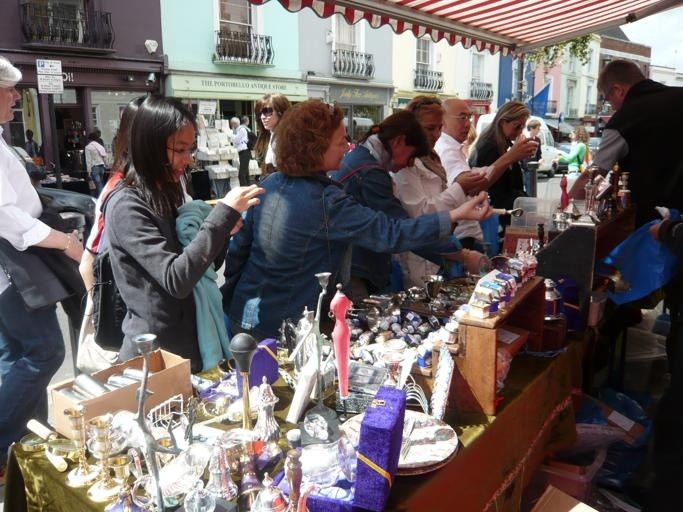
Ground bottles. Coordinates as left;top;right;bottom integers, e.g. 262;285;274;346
585;168;598;213
545;276;562;322
56;316;337;511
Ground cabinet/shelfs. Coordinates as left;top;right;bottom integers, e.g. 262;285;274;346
12;196;636;512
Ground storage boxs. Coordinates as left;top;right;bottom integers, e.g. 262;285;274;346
621;323;669;395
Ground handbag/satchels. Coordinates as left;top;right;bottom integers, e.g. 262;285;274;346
90;182;134;352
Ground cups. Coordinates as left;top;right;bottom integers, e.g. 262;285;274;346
618;189;630;209
526;141;540;159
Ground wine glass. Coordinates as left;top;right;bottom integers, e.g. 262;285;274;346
420;274;446;313
377;336;408;386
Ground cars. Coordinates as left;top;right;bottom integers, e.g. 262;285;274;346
34;185;98;242
475;114;608;177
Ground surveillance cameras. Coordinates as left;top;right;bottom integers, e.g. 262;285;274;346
147;73;156;84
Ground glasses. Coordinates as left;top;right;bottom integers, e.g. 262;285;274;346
510;120;526;131
166;146;198;161
259;106;281;117
